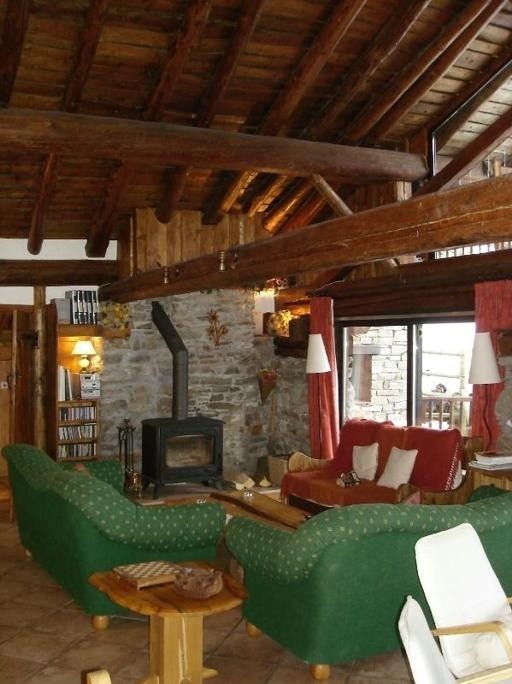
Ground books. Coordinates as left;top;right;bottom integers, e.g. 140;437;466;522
336;471;361;488
59;406;97;458
112;560;183;591
468;451;512;471
58;365;73;401
65;290;96;324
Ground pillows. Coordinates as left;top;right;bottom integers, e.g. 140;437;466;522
352;442;380;481
375;446;419;490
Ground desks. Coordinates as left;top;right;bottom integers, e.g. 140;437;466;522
86;560;246;684
165;488;315;533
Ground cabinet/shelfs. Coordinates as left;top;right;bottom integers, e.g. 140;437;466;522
45;303;104;464
466;463;512;491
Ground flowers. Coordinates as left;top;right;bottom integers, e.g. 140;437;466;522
256;368;281;402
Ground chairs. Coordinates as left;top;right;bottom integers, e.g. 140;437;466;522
398;593;512;684
415;523;512;679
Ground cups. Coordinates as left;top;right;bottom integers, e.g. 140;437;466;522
242;487;255;505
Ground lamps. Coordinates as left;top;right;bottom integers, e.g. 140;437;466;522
71;340;96;374
468;331;512;464
161;265;181;284
306;333;332;460
217;249;238;273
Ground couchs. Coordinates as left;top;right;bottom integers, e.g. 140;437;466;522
1;442;225;628
281;418;483;508
225;485;511;681
